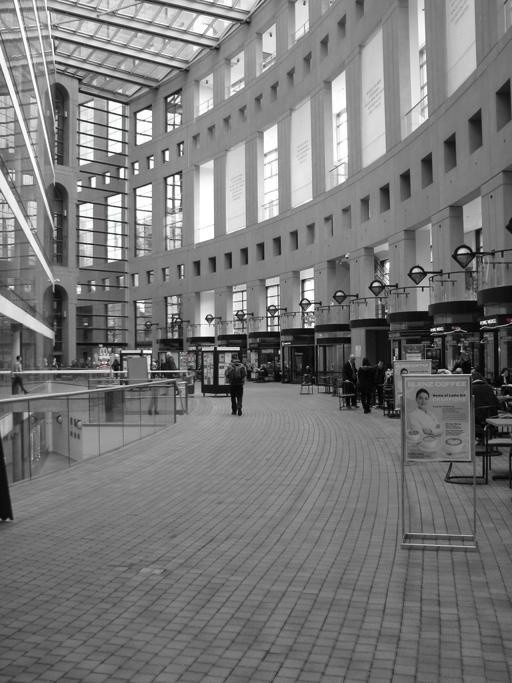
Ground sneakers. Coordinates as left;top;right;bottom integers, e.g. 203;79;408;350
345;404;360;411
231;407;243;416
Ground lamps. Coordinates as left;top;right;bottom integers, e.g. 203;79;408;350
144;321;159;329
266;290;370;318
174;310;264;327
367;244;512;298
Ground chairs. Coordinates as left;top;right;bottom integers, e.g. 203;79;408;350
295;359;511;487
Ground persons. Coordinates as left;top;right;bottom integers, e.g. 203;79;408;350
224;355;247;415
163;350;180;395
410;387;438;432
357;356;376;414
147;358;161;415
260;365;268;376
112;358;121;379
371;359;385;407
342;354;359;408
454;365;511;446
11;354;30;394
395;367;409;394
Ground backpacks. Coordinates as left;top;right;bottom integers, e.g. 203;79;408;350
228;362;242;380
